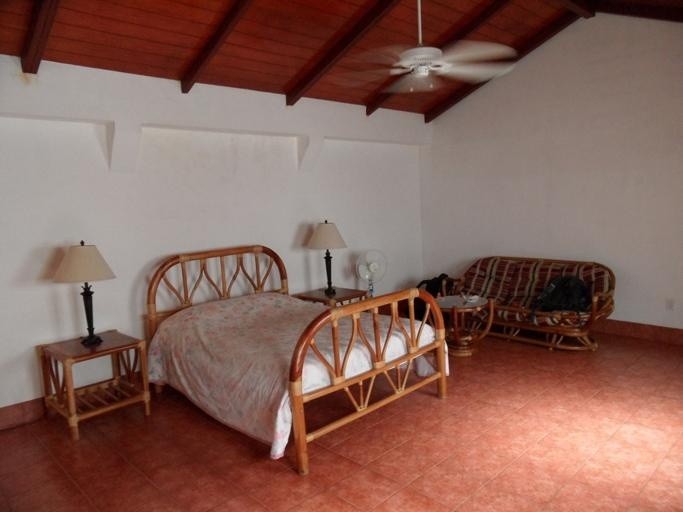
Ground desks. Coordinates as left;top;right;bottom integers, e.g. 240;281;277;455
34;327;153;441
289;286;369;308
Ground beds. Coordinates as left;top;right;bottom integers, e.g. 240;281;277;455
142;243;453;478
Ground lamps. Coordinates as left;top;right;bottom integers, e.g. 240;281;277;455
51;239;118;346
305;219;350;294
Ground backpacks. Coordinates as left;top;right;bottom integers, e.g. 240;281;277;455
530;275;589;326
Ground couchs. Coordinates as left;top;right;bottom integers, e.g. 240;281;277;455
439;254;618;353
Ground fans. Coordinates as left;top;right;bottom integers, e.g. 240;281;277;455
322;3;518;95
354;246;388;300
